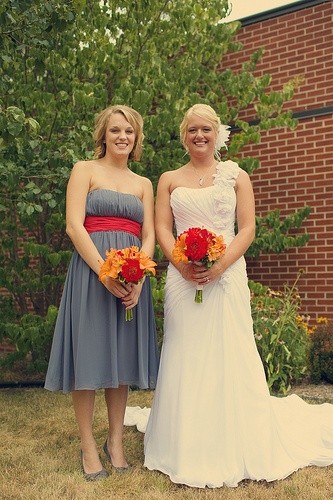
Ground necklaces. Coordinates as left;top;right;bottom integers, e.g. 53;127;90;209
187;161;218;186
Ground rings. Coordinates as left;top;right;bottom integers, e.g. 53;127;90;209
205;277;208;281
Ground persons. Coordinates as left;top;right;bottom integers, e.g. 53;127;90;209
44;103;158;480
144;103;271;490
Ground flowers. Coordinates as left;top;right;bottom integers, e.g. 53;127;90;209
172;228;227;304
97;246;157;322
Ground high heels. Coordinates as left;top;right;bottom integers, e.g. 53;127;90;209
104;442;129;472
80;446;108;482
